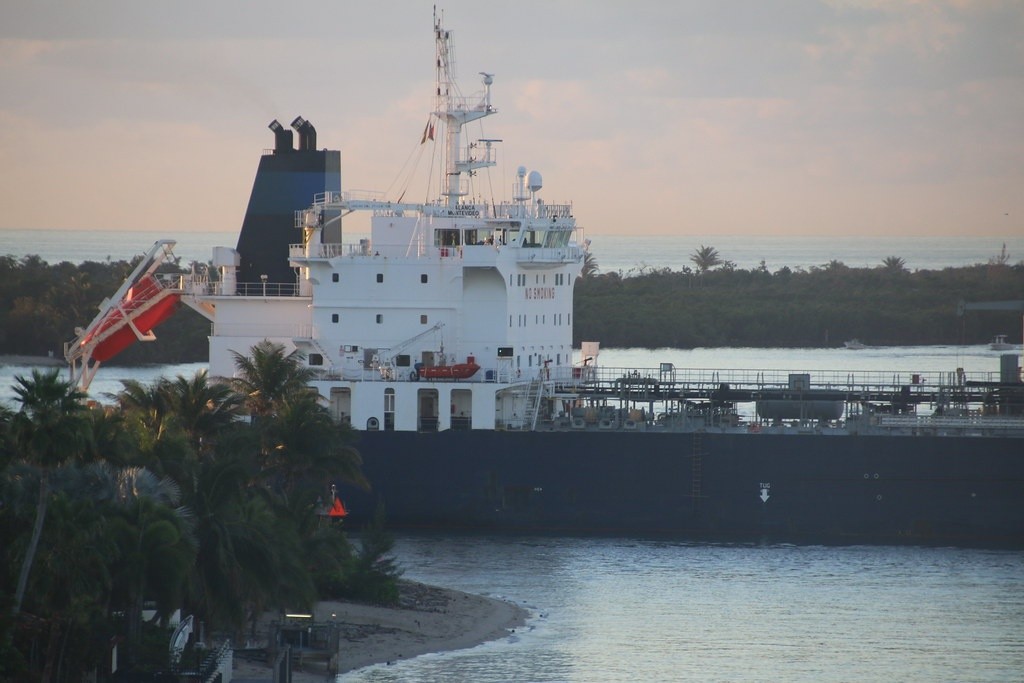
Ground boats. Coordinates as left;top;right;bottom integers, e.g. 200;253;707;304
844;339;864;349
62;3;1023;546
990;336;1016;351
